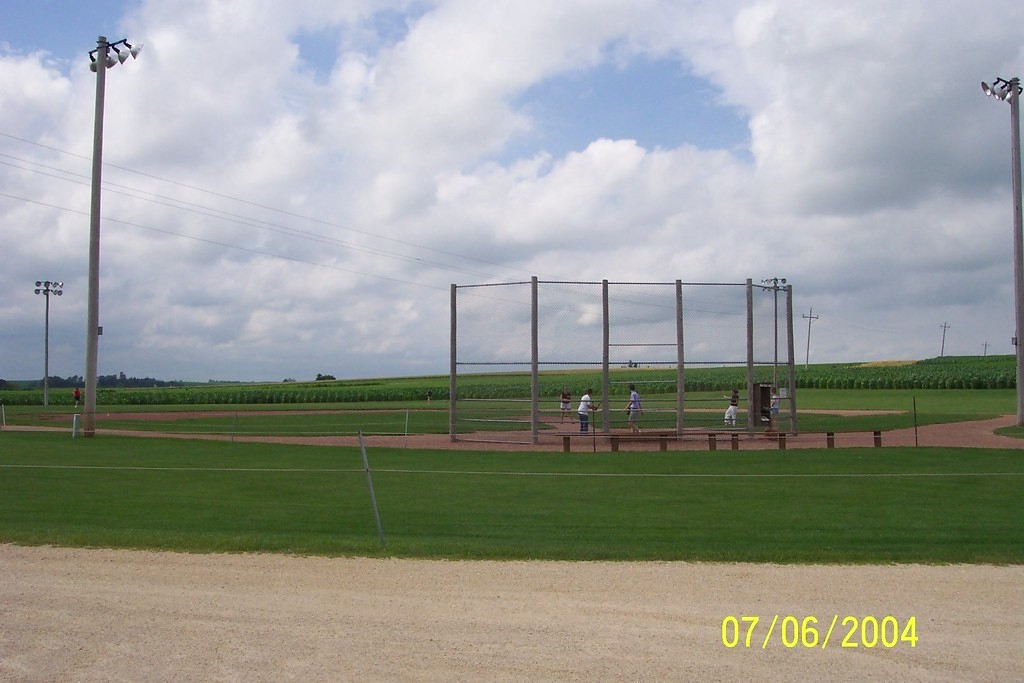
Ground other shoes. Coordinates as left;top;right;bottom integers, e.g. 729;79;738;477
764;428;772;432
770;428;779;432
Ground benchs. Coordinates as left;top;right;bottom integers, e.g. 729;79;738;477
554;429;888;452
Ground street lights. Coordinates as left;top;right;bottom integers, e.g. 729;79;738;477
83;34;145;439
34;280;64;406
982;76;1024;428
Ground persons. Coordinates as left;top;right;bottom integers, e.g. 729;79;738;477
560;386;575;424
578;389;597;432
72;387;81;408
770;386;779;431
724;388;739;426
624;383;643;432
